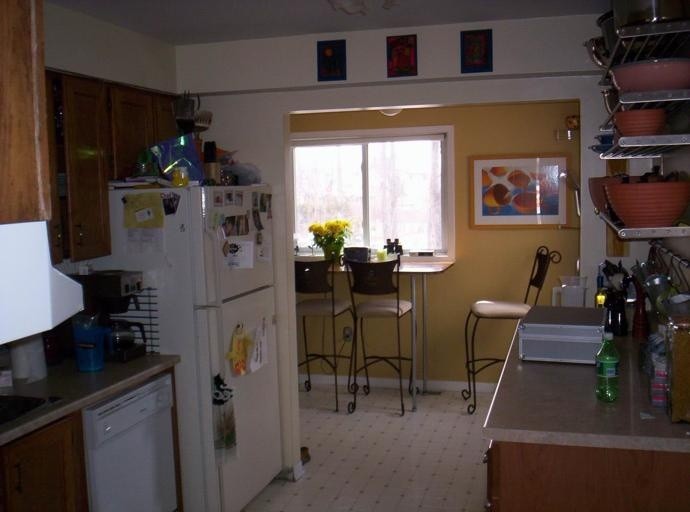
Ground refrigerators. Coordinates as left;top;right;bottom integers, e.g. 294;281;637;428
109;178;284;511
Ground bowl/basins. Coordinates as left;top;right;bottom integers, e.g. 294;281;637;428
612;111;668;136
587;174;689;227
588;134;613;152
583;0;689;115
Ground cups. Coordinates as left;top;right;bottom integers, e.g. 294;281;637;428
376;250;387;261
641;270;690;314
204;162;221;184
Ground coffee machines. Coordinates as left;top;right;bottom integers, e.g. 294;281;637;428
92;270;146;362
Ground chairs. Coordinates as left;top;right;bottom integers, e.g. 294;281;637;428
459;245;563;415
295;253;416;416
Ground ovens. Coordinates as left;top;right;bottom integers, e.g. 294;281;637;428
79;374;179;512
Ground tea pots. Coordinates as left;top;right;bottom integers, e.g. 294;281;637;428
552;275;588;308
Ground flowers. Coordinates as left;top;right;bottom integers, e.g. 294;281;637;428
308;218;351;247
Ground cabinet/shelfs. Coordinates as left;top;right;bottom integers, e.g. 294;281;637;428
109;82;175;185
482;439;688;512
42;71;111;266
1;410;91;512
598;20;689;242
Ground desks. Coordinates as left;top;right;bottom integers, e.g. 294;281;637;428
296;258;455;394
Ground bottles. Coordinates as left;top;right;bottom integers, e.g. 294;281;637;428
594;333;620;403
595;277;628;334
172;166;188;187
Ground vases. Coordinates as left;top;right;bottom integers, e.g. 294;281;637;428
323;241;343;265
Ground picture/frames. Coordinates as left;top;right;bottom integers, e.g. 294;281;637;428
468;151;571;229
